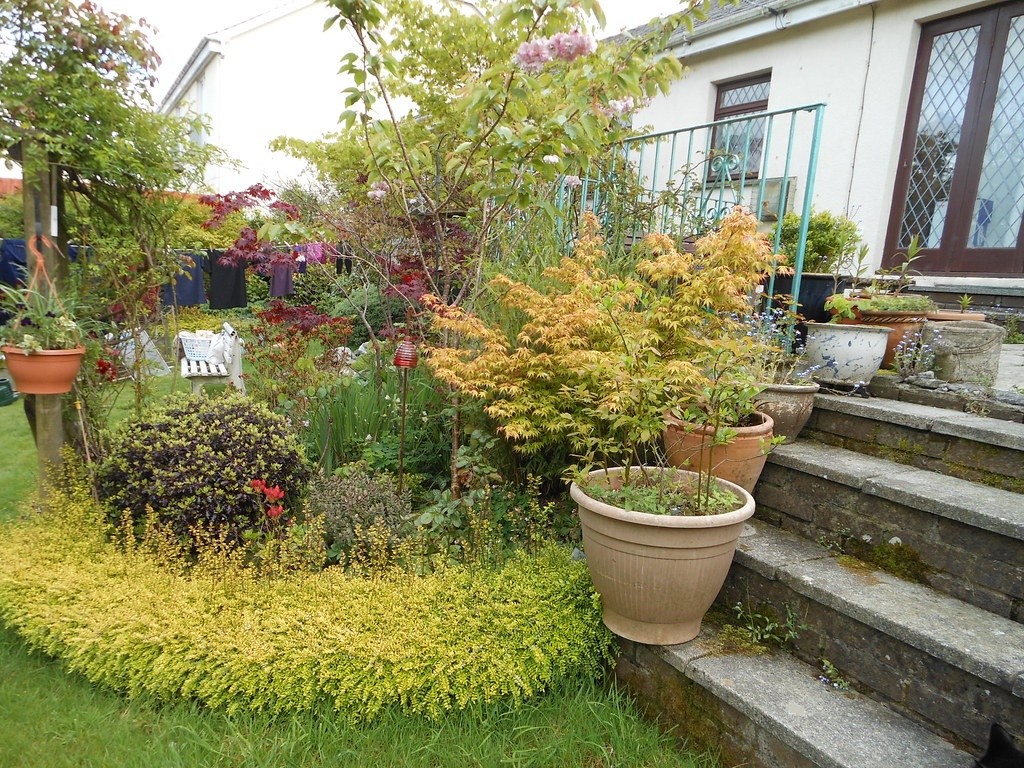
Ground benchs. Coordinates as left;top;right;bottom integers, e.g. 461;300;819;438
179;322;246;396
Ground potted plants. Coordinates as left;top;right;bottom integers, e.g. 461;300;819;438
422;207;985;646
0;314;85;395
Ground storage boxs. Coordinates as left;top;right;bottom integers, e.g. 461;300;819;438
182;336;210;362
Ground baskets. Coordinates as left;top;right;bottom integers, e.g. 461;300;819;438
179;331;212;360
